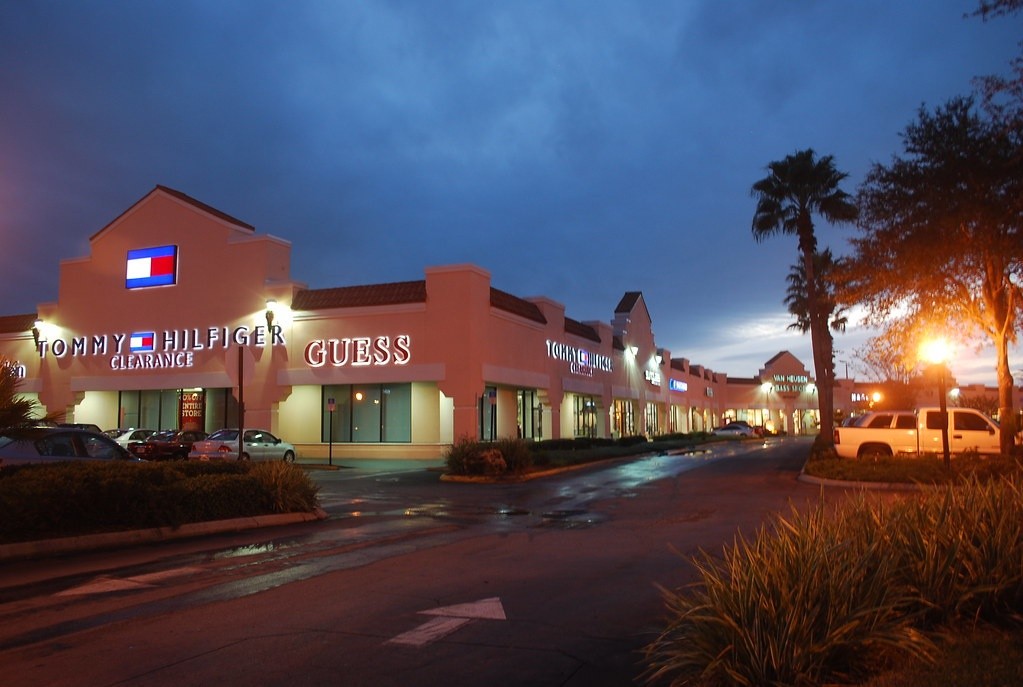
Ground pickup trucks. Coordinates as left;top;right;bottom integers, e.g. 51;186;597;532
833;405;1023;465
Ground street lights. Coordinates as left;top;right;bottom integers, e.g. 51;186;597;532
919;335;954;468
838;359;849;380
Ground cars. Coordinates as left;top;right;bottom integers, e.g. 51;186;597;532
841;408;918;428
709;423;755;439
84;426;157;458
0;425;149;466
186;427;297;465
127;427;211;462
55;421;102;434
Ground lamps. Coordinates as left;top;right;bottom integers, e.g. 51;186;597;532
266;298;277;333
31;319;47;347
